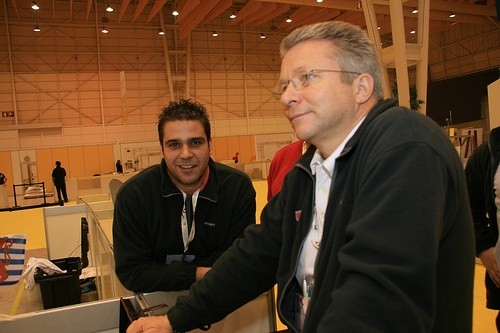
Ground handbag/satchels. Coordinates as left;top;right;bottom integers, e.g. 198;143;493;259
0;234;26;286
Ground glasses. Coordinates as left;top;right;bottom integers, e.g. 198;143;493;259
272;68;362;100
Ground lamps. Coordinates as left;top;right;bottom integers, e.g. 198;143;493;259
212;31;219;37
158;0;180;35
102;1;114;33
31;0;42;32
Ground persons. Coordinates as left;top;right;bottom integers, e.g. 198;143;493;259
0;173;9;209
267;139;310;202
112;98;257;333
126;20;474;333
453;130;477;158
116;160;123;174
465;126;500;333
52;161;69;206
235;151;239;163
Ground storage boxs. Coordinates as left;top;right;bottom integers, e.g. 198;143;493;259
36;216;89;310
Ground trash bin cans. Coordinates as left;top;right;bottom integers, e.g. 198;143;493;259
34;257;82;309
